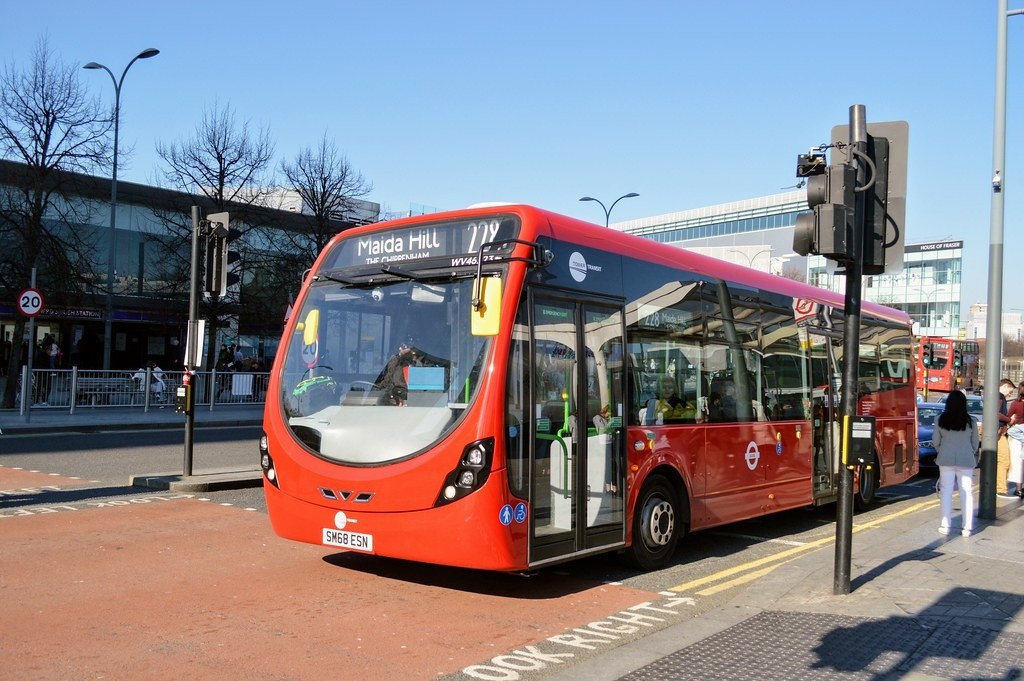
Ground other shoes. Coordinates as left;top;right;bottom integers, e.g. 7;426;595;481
962;529;971;538
155;394;160;400
159;405;165;408
43;401;50;407
938;527;951;535
1014;488;1024;500
997;492;1019;499
50;373;57;376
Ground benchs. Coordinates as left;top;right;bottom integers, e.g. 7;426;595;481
76;378;180;410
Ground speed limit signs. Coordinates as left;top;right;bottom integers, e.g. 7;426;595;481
17;288;44;316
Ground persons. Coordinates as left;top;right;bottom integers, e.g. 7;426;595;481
668;361;676;375
933;390;979;537
639;399;663;425
813;403;826;472
382;333;424;399
765;396;792;419
593;404;611;434
568;411;577;454
650;359;655;370
708;391;722;418
216;343;261;402
998;379;1024;499
656;376;686;409
33;333;58;406
136;361;168;409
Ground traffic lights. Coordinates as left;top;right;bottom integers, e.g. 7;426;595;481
207;212;242;298
954;349;961;366
922;344;931;365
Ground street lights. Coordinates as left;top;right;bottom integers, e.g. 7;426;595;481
82;47;159;376
579;193;640;228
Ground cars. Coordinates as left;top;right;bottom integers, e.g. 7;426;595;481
917;402;946;469
936;394;983;441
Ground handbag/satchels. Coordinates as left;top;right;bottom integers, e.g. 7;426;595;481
978;425;983;441
45;346;51;356
227;362;234;367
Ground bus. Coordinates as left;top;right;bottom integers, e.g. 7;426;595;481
260;205;919;577
916;337;979;391
